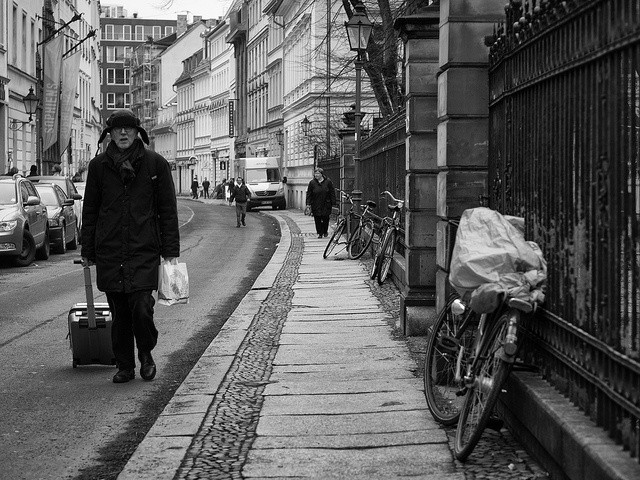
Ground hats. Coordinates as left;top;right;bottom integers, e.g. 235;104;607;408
98;108;150;146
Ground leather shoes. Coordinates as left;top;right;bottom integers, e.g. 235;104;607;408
140;353;156;380
113;369;134;383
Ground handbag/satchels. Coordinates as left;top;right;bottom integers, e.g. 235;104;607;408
158;258;190;307
304;205;312;216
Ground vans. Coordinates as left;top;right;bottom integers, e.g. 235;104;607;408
231;157;288;212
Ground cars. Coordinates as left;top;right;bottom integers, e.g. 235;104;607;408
0;173;50;267
73;180;87;204
27;172;86;253
30;182;79;255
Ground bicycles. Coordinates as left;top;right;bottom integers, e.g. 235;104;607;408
368;190;405;286
420;203;550;461
322;187;377;262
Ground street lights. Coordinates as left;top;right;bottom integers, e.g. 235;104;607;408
59;27;100;165
342;2;376;265
33;9;84;171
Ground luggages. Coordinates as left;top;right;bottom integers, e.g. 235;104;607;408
68;260;117;368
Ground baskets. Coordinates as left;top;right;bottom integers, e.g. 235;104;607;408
448;219;461;274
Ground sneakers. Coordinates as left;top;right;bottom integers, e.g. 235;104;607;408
241;220;245;226
318;235;322;238
237;224;240;227
324;233;328;237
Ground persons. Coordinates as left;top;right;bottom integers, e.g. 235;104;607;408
305;167;336;239
72;171;83;182
202;177;210;199
6;167;19;176
26;164;38;177
230;177;251;228
51;162;63;177
190;178;199;199
80;109;181;383
